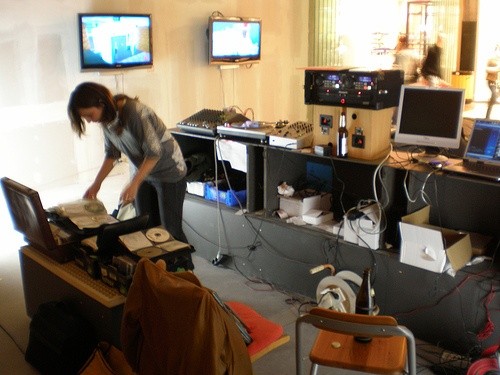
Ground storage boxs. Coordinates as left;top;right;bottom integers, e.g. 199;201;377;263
302;208;334;225
186;170;230;197
277;187;331;219
343;202;383;251
204;182;247;208
398;204;472;274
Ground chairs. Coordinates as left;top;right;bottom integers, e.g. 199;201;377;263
295;307;416;375
119;256;291;375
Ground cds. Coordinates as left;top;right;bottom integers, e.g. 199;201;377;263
147;228;169;242
138;247;161;259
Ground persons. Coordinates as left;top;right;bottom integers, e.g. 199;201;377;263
68;81;195;274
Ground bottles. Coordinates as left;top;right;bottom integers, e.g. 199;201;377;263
336;112;349;158
354;270;373;344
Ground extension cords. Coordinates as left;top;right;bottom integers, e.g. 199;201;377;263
440;352;473;369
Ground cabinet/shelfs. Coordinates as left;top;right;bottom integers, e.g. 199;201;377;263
169;123;500;358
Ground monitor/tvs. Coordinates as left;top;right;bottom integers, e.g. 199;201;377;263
209;17;263;64
463;120;500;170
394;86;465;162
77;13;154;72
